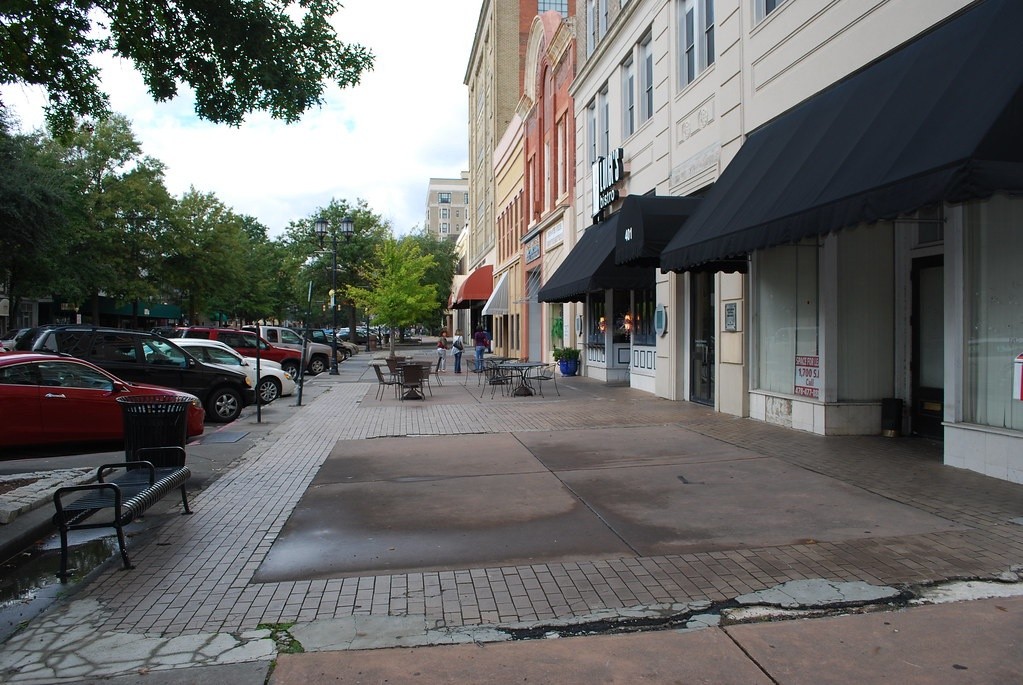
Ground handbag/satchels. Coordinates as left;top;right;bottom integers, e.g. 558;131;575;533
437;337;444;349
484;339;487;347
453;336;463;350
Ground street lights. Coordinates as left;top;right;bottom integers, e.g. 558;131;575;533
314;217;355;375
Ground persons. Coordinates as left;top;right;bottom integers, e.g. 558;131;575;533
436;330;449;373
474;326;491;373
451;329;465;374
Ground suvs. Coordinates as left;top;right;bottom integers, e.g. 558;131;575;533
11;323;256;424
166;325;302;382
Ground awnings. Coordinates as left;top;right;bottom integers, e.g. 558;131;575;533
538;211;620;303
448;265;494;309
615;194;703;263
481;272;507;316
75;295;184;321
660;0;1023;276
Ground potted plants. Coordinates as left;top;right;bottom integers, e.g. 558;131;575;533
553;347;581;378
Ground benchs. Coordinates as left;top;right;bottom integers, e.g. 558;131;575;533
55;446;194;585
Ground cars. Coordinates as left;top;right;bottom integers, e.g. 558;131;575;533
284;326;359;360
0;350;206;447
336;326;383;345
125;337;295;405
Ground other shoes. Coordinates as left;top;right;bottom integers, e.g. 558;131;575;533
438;369;445;372
475;369;483;373
455;370;461;373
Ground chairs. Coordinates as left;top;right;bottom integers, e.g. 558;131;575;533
464;356;560;402
372;357;442;403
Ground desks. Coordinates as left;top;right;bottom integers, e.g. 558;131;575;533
476;357;520;376
498;361;549;397
395;367;429;400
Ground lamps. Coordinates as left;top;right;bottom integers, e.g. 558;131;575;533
623;312;632;336
598;316;607;333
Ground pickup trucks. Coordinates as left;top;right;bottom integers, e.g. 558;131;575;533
241;324;342;376
